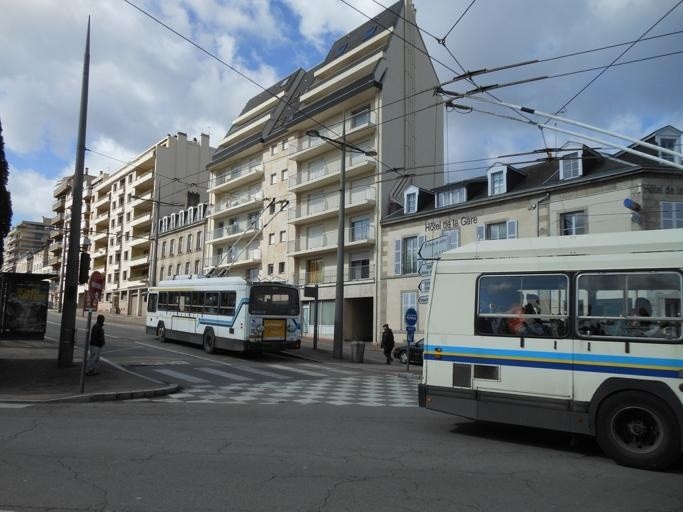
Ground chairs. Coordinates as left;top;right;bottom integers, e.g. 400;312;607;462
549;313;564;338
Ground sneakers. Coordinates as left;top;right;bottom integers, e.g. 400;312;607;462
85;371;99;377
384;358;394;365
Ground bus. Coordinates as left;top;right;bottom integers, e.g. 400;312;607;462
414;223;682;476
141;274;303;358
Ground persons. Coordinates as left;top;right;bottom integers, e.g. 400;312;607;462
488;289;670;338
378;321;398;365
85;313;106;378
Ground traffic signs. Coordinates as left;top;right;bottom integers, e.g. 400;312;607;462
416;233;450;306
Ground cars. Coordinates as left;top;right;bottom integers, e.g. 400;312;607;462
393;336;423;365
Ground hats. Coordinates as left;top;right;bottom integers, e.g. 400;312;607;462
382;324;388;327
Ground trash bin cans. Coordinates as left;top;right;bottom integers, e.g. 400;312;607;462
351;342;365;363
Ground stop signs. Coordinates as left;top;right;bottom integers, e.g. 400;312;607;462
87;270;103;301
